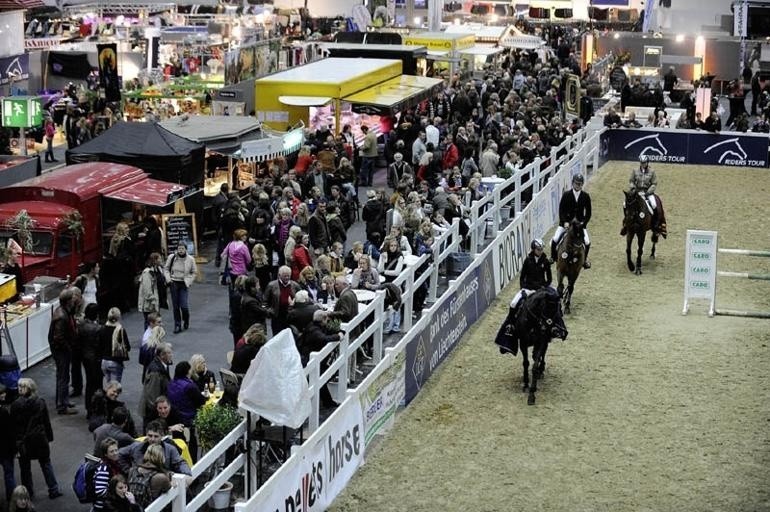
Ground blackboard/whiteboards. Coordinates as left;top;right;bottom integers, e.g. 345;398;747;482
161;213;199;258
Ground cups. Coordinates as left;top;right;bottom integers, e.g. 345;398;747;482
205;171;228;193
317;293;337;312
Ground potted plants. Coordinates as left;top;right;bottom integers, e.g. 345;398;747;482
493;165;515;220
193;402;244;510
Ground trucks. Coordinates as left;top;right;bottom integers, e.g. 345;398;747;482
1;160;205;299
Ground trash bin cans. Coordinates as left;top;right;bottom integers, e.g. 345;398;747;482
478;176;505;193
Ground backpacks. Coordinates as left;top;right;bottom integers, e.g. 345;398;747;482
128;467;157;507
72;457;98;504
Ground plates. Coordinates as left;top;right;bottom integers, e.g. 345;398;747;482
353;290;365;296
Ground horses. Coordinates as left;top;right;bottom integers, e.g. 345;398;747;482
555;220;586;315
517;287;569;405
622;189;665;276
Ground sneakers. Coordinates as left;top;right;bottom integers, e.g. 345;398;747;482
49;491;63;499
57;391;83;414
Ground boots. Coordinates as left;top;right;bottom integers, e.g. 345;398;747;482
548;240;556;264
583;243;591;268
182;308;190;329
173;309;181;333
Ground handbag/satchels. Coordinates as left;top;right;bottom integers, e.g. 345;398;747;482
115;329;130;362
220;266;232;286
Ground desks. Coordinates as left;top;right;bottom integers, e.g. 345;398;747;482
478;176;506;226
314;241;427;384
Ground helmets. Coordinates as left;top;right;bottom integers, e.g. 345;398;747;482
573;173;584;185
532;238;545;250
640;155;649;163
